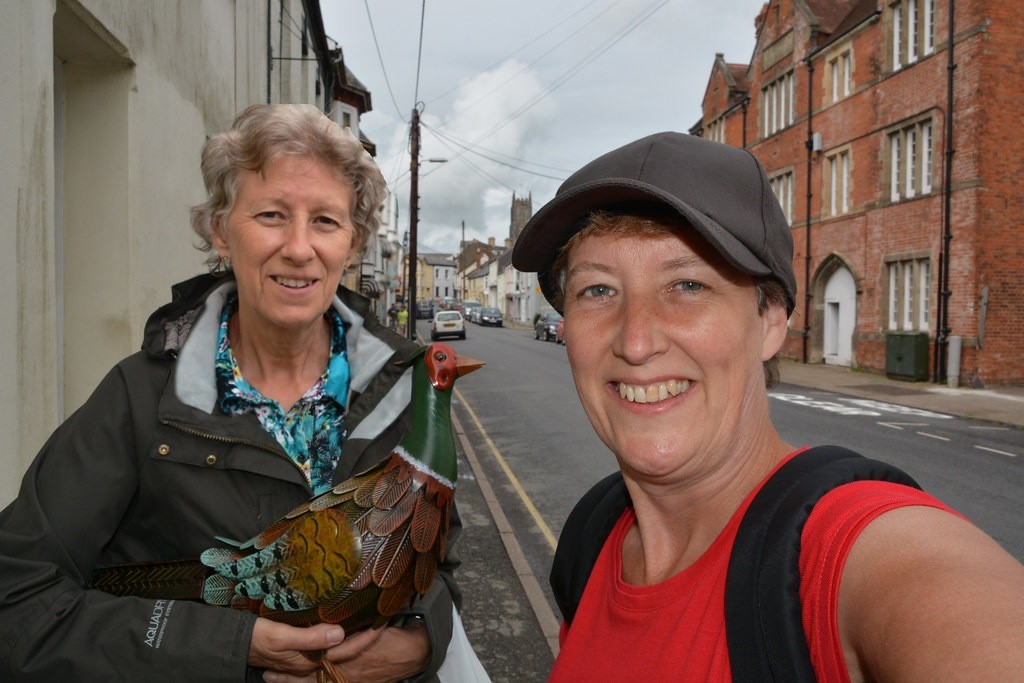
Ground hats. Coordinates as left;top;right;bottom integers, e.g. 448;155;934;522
510;129;797;319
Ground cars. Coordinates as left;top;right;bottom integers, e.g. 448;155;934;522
534;311;563;342
428;310;467;342
555;317;566;346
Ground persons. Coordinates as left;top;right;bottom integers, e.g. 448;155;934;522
388;304;399;332
0;103;465;683
398;305;408;337
511;132;1023;683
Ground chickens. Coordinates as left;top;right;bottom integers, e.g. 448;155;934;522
85;343;486;683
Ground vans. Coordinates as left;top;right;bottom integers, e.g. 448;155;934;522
461;299;481;320
480;307;504;328
470;307;481;324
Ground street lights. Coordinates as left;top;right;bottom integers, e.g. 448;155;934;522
408;158;450;341
